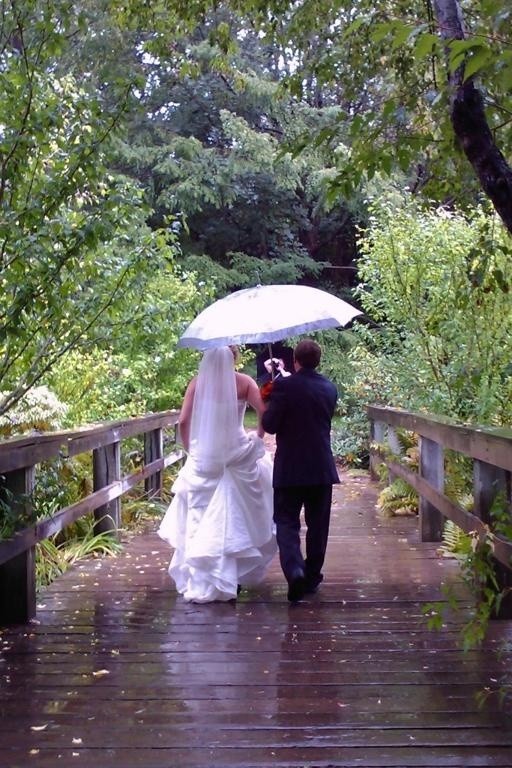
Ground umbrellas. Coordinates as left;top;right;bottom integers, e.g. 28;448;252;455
177;271;365;348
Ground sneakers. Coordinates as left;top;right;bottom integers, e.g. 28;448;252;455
288;571;323;604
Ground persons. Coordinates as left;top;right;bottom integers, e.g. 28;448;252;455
257;340;342;602
156;346;278;605
264;357;293;382
